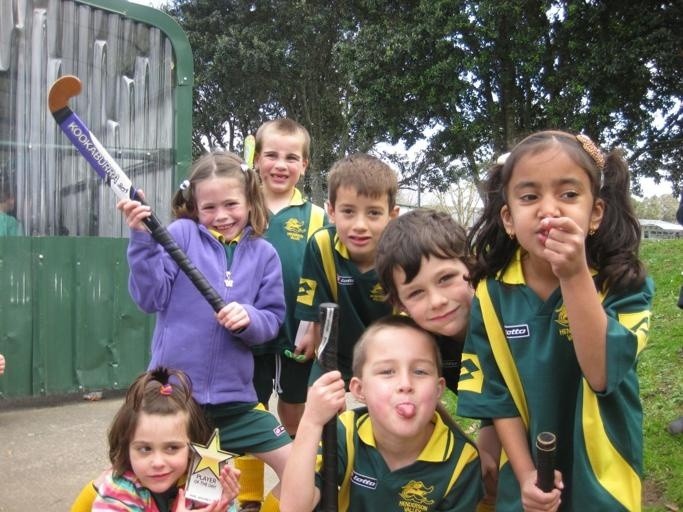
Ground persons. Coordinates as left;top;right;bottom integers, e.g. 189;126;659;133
666;193;683;435
278;313;489;512
373;208;502;512
454;129;656;512
233;115;332;512
90;363;242;512
292;154;401;396
0;354;6;375
71;150;293;512
0;188;24;237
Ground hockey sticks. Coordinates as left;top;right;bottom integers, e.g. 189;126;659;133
48;75;224;310
